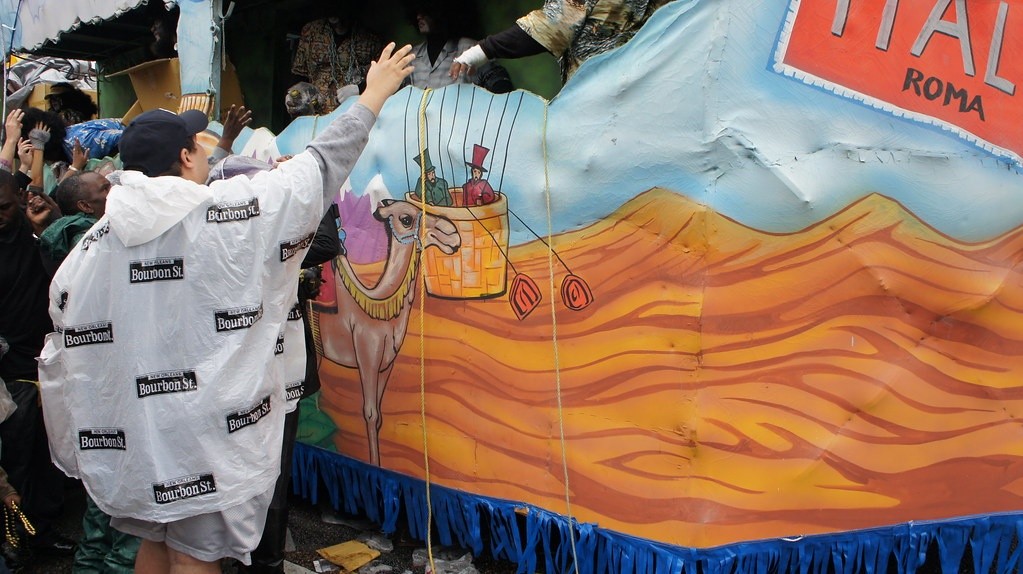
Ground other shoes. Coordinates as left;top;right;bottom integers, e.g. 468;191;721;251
49;536;79;557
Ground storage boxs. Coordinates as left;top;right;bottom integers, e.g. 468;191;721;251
29;84;98;119
105;57;245;127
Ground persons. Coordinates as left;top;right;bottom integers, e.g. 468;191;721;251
448;0;676;88
37;40;417;574
0;83;343;574
285;1;389;119
401;0;515;93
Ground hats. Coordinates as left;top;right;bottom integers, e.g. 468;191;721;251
117;109;209;178
44;81;71;100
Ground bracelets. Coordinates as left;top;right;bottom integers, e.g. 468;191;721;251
67;165;78;171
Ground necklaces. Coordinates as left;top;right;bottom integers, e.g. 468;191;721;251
326;21;360;88
3;499;36;548
